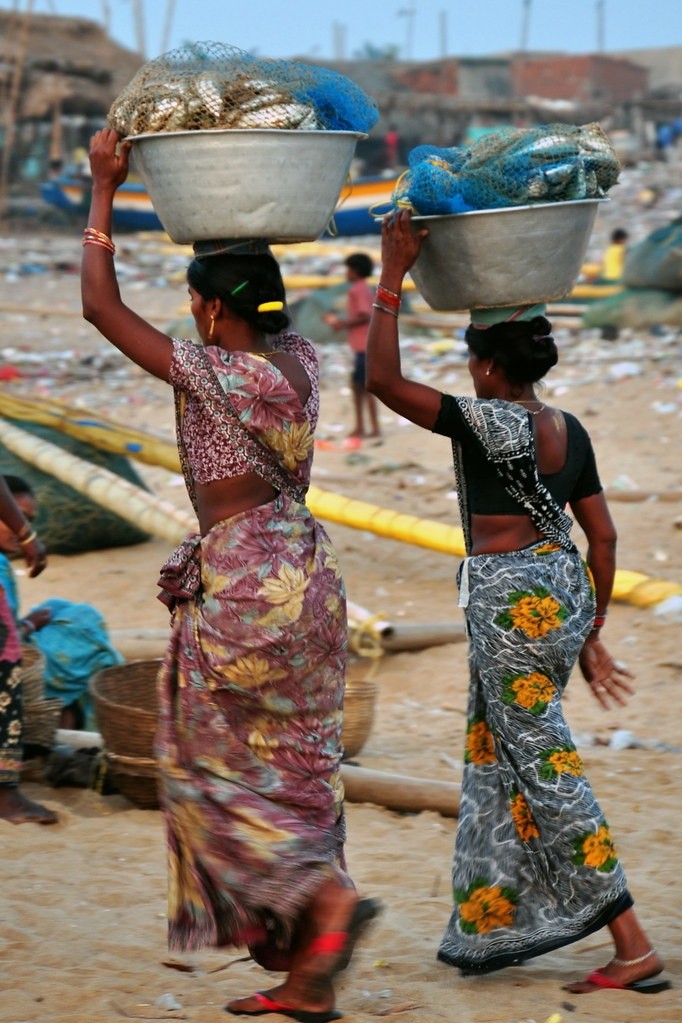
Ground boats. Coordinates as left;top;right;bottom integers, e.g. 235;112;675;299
38;159;414;240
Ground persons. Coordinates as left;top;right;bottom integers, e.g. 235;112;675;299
323;253;383;438
76;125;388;1023
599;228;628;281
365;205;671;998
0;474;83;783
0;458;60;826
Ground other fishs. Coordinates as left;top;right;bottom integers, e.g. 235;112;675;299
114;68;327;137
452;117;622;199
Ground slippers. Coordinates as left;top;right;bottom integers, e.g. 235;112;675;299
222;992;342;1023
308;896;378;974
563;967;671;995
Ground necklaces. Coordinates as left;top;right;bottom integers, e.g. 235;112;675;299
513;398;547;416
252;351;283;359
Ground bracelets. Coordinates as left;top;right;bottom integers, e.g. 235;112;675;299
81;227;116;256
592;609;607;630
372;284;401;318
16;519;40;548
22;619;37;631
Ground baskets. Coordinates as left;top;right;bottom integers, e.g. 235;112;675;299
87;660;169;809
15;697;62;778
339;678;376;760
17;643;46;703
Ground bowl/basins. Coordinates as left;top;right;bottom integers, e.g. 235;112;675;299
374;197;611;312
121;129;369;245
